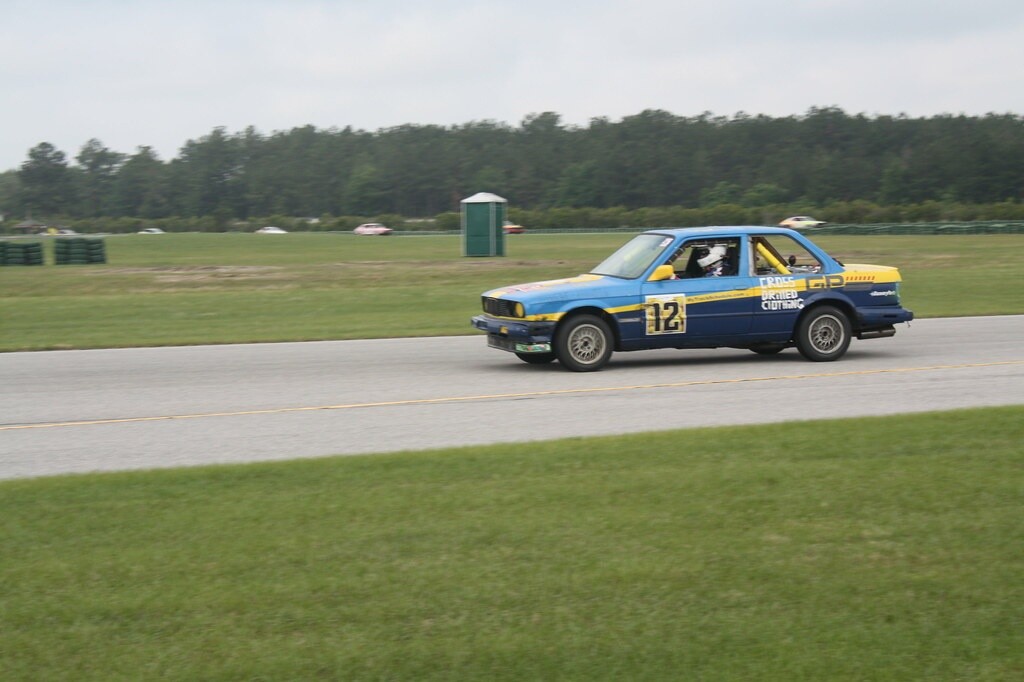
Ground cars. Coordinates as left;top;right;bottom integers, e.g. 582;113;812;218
354;222;393;235
470;225;916;374
254;227;288;234
503;221;524;235
137;227;165;235
778;216;828;229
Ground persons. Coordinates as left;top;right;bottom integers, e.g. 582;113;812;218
670;243;728;279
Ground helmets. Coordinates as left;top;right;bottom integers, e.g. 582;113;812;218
691;238;727;268
